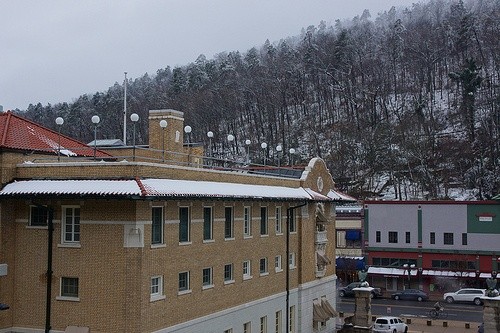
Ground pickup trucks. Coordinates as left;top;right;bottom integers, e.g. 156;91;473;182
337;281;384;299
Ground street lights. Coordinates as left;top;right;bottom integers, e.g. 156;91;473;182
207;131;214;166
55;116;64;162
130;113;139;161
91;115;100;161
276;145;283;174
245;140;251;165
159;120;167;163
289;148;295;169
184;125;192;166
227;135;234;172
261;142;268;175
403;263;415;289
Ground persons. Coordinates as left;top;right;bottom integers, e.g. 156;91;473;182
434;301;442;317
478;323;484;333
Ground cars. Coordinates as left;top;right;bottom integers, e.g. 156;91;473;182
391;289;429;302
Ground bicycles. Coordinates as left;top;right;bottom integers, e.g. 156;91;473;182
430;308;448;317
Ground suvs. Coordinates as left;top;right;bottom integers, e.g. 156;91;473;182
372;317;408;333
443;288;488;306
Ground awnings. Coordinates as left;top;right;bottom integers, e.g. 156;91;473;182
336;255;365;271
